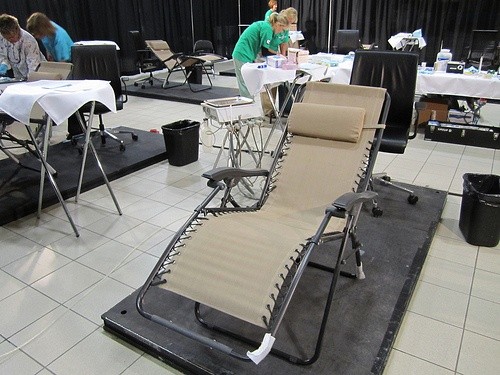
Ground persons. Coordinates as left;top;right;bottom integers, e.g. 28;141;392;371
264;0;279;21
232;7;298;118
26;13;88;141
0;13;47;81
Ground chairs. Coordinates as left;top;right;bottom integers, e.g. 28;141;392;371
333;29;361;53
27;60;73;82
146;40;229;94
134;78;396;366
68;43;138;152
195;40;219;79
134;49;169;89
350;49;422;215
464;29;500;72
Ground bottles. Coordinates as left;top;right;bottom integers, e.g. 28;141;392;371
437;49;453;62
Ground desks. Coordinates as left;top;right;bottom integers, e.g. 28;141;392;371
388;32;428;53
0;78;123;237
412;67;500;125
240;60;334;155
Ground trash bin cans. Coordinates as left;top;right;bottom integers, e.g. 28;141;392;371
161;120;200;167
185;66;202;84
459;173;500;247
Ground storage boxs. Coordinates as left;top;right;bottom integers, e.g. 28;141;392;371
286;47;309;65
446;61;466;74
415;101;449;126
267;55;285;69
